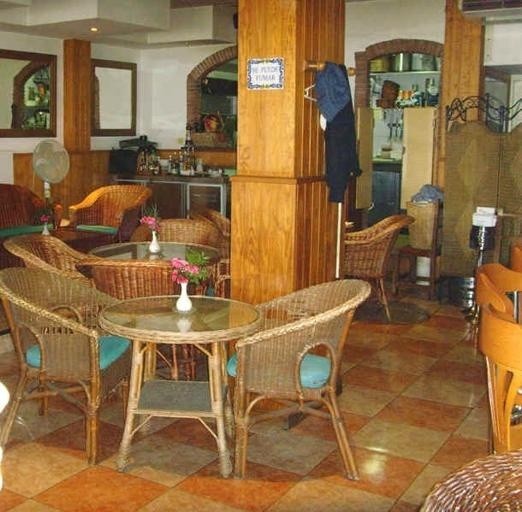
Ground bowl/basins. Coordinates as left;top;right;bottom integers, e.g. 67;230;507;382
375;79;400;108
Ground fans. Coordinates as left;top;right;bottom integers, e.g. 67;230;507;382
32;139;70;199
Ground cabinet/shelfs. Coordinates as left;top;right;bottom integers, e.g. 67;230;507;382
350;38;444;238
113;170;229;219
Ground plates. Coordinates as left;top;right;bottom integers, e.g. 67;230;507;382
369;59;390;73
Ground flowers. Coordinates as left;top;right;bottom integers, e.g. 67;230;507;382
170;248;210;284
141;215;160;232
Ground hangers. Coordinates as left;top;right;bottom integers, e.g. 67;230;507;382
304;62;320;102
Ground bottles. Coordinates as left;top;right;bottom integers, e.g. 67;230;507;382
138;136;223;176
398;78;438;108
382;144;391;159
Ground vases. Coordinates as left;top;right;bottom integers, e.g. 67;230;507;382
149;230;160;253
176;282;192;311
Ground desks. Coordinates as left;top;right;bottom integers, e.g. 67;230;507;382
56;219;77;242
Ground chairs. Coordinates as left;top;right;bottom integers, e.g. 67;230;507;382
227;278;373;481
391;203;443;300
420;450;522;512
214;257;230;296
474;238;522;456
344;214;416;322
0;267;137;466
67;186;152;248
74;257;196;300
194;208;230;256
4;233;86;272
131;220;219;249
0;184;64;236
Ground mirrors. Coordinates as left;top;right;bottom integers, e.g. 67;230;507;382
0;48;57;138
91;58;137;136
